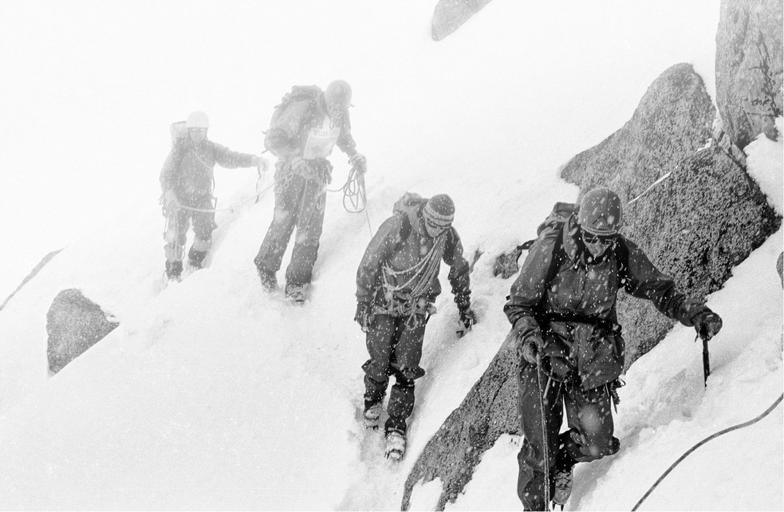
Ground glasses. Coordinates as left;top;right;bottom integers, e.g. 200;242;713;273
425;217;453;230
582;230;622;246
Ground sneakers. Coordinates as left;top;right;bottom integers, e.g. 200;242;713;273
261;273;279;294
364;398;380;421
551;461;572;509
284;284;303;308
385;423;403;463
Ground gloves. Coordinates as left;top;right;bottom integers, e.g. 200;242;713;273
460;316;476;332
353;303;373;333
695;311;723;340
521;338;545;366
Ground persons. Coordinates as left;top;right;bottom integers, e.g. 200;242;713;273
354;194;476;460
504;188;722;511
160;111;266;286
253;80;366;301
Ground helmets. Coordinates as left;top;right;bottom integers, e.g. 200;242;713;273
576;188;624;237
327;81;350;102
188;112;207;129
425;193;456;226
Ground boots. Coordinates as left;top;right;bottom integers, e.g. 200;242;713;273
163;246;208;281
